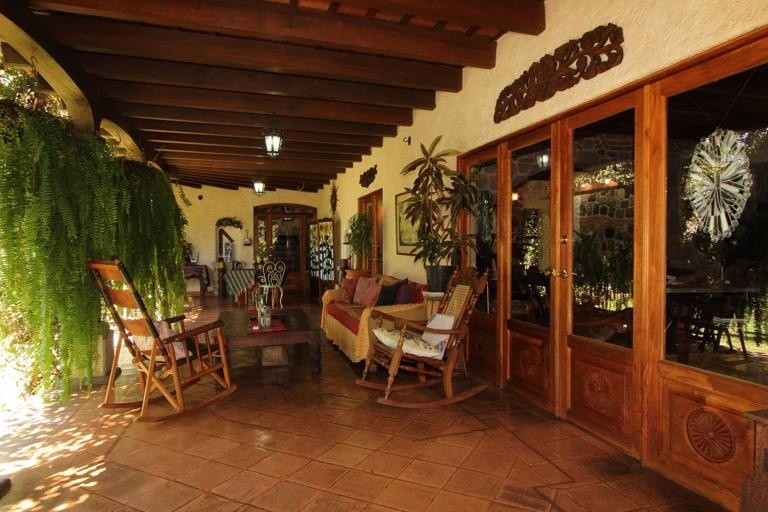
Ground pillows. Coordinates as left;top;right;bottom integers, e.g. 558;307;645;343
336;270;429;307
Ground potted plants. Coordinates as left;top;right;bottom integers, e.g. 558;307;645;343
400;134;494;291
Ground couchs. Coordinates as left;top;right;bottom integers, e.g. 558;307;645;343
321;274;430;364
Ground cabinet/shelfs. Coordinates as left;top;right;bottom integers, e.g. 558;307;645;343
254;203;317;294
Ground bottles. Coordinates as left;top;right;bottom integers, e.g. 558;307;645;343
254;284;261;309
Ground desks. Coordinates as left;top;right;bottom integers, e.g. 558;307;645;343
228;268;257;305
184;265;207;306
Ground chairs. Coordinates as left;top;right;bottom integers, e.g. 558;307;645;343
355;266;489;409
255;261;286;309
85;260;238;423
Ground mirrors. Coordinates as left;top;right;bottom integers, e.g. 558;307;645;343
272;217;303;286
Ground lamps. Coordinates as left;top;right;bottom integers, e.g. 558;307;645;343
259;111;288;157
253;168;266;196
